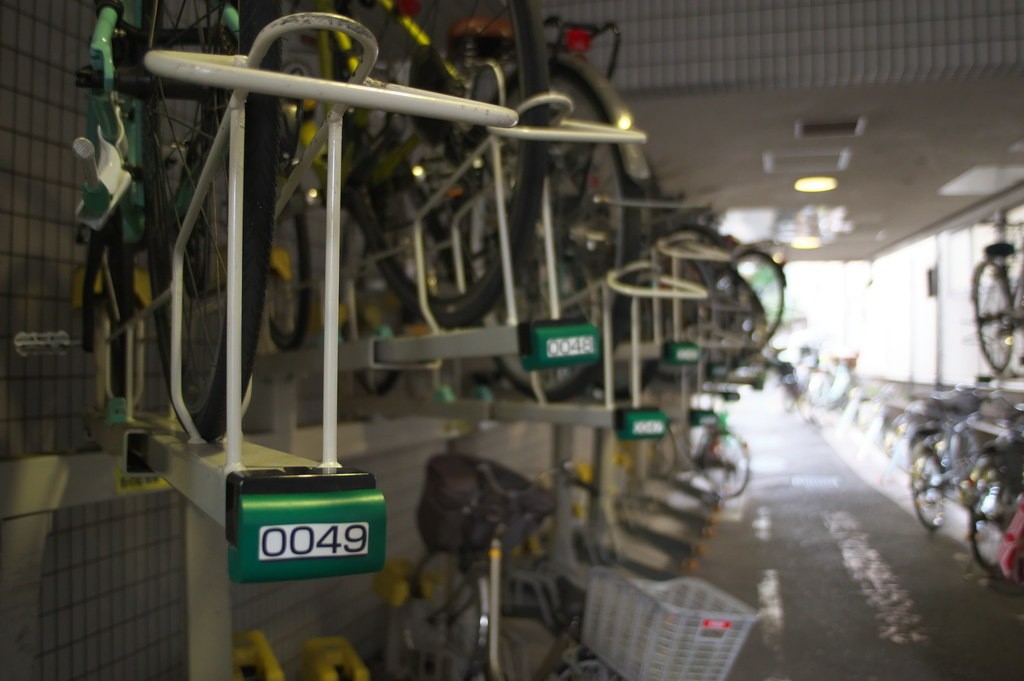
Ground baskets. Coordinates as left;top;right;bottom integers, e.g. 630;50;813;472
579;566;762;681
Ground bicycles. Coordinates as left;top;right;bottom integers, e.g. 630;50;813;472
653;420;750;498
382;437;758;681
73;1;786;446
901;217;1024;591
